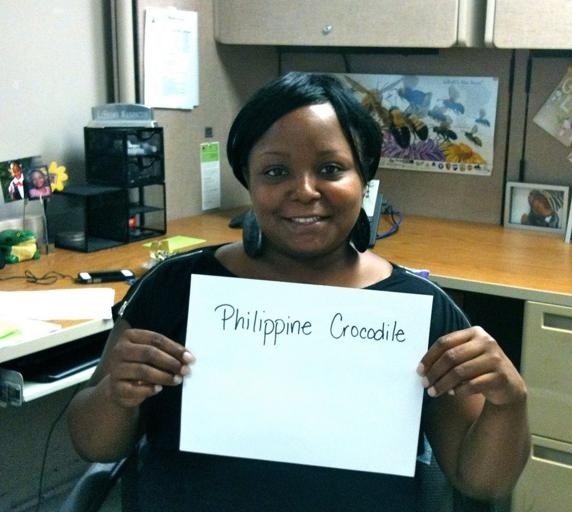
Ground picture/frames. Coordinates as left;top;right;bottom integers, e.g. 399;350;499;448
501;179;572;237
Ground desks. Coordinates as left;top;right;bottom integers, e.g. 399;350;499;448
0;207;571;512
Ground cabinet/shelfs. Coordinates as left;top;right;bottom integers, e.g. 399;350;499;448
482;1;572;51
47;125;169;254
214;0;484;49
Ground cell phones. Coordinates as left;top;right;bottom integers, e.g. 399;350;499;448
78;270;134;283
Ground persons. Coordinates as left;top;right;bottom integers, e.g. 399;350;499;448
7;161;28;201
521;191;559;228
67;74;533;512
27;169;51;198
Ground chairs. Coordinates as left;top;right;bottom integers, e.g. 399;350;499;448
55;270;454;512
